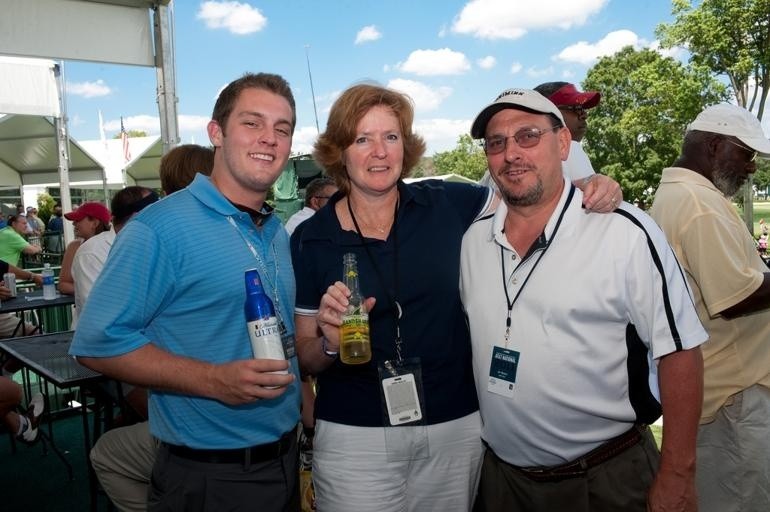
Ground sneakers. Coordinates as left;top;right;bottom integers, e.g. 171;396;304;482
16;393;49;447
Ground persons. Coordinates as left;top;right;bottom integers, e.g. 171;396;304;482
0;73;768;512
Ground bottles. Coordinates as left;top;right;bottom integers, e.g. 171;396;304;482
340;252;372;365
42;264;56;300
245;269;289;390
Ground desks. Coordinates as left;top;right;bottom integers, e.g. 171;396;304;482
0;329;118;510
0;276;95;423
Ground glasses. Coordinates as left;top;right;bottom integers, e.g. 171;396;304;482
722;137;761;163
554;103;587;115
479;122;562;156
312;193;333;201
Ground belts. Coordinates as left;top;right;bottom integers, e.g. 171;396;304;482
154;429;299;466
484;421;650;487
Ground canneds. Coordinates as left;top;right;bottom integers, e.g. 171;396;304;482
4;272;17;297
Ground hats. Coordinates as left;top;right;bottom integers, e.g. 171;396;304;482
683;102;770;162
60;199;112;229
471;87;568;131
530;79;601;116
